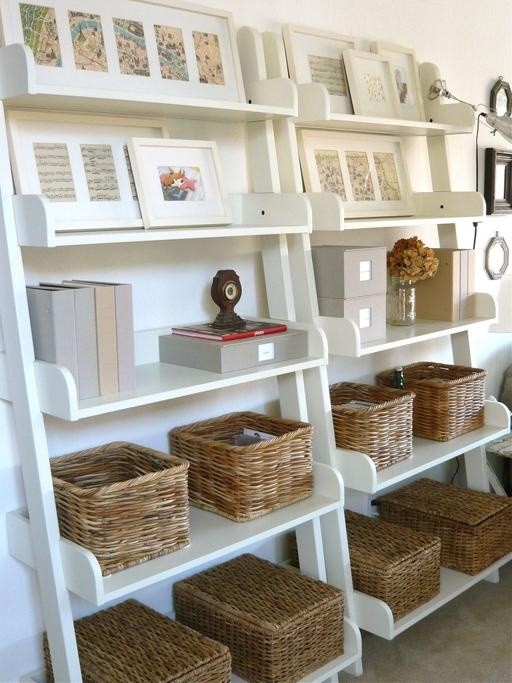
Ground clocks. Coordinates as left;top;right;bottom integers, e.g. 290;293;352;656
210;268;246;330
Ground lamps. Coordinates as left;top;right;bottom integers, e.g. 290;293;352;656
471;112;512;247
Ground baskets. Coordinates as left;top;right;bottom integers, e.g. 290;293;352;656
42;362;512;683
327;380;415;472
44;598;234;683
375;362;489;442
371;477;511;576
48;439;191;575
171;553;345;683
286;509;444;622
166;409;314;525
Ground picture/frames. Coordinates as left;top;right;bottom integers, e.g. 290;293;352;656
2;0;247;106
284;28;357;117
490;76;512;117
342;49;404;119
485;148;512;215
298;128;417;220
126;136;232;229
485;237;509;280
5;109;170;234
370;41;426;121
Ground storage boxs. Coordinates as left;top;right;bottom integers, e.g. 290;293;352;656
311;245;386;343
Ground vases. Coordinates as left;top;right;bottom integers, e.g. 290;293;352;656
387;276;417;326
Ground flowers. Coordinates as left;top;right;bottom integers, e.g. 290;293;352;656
385;235;440;283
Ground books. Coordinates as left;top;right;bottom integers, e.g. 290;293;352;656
413;245;460;321
460;248;467;321
467;249;476;318
170;319;287;341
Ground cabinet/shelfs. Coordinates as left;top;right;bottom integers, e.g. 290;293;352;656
261;30;512;677
0;0;363;683
